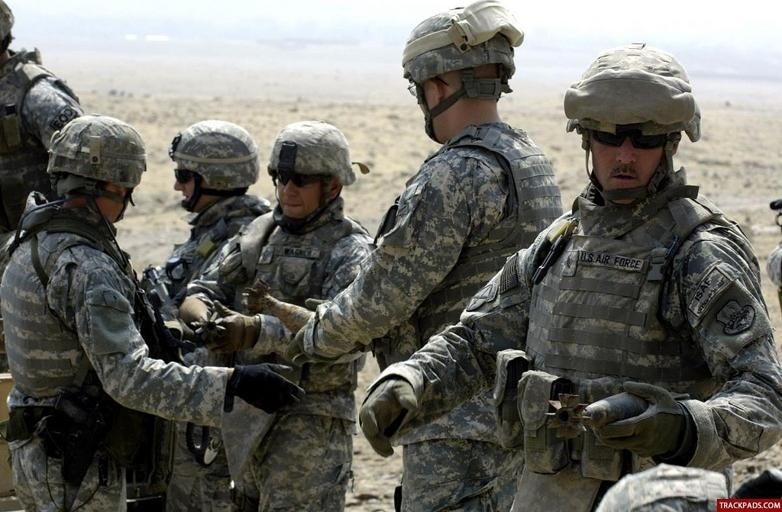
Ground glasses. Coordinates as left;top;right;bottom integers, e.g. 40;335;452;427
171;165;193;184
405;79;417;96
274;170;327;188
590;124;670;151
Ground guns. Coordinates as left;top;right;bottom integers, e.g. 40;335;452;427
138;264;183;363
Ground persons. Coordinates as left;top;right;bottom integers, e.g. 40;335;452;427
169;122;375;511
136;120;274;507
359;40;781;511
0;0;87;374
283;0;564;509
2;114;308;510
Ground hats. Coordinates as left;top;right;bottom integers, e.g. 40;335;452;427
266;120;356;188
402;0;519;96
563;43;702;144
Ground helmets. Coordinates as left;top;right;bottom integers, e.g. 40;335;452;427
167;119;260;190
45;114;149;189
0;0;15;45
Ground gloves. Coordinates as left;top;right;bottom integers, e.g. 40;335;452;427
285;298;327;367
226;363;306;416
358;376;425;458
591;378;688;458
202;300;264;356
